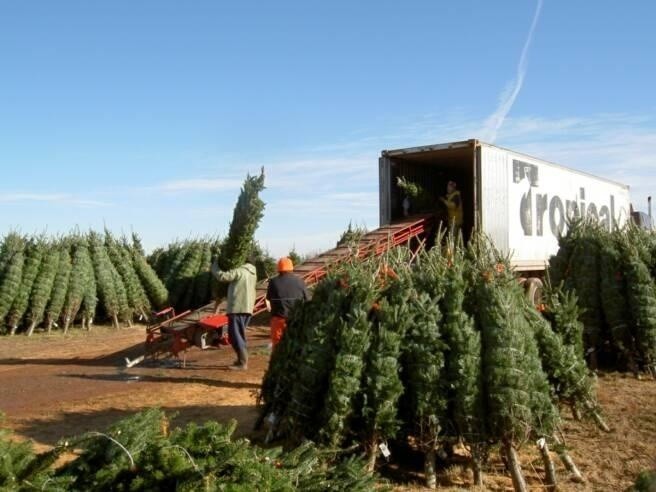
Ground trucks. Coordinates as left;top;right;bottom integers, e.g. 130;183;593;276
377;139;654;311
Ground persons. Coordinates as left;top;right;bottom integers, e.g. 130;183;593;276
266;256;313;352
209;254;258;370
439;181;463;240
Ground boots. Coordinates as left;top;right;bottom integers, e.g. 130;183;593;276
229;349;248;370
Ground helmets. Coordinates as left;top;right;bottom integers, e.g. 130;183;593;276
278;257;293;272
